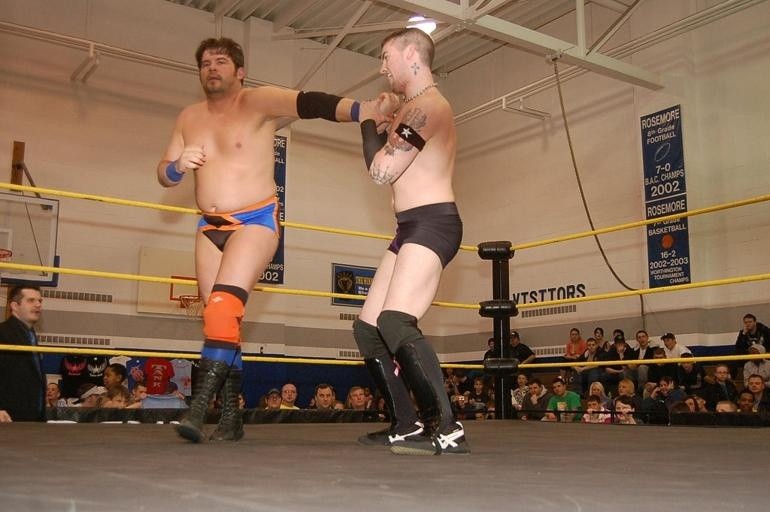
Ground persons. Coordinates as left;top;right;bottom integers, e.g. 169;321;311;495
158;38;398;441
358;27;472;454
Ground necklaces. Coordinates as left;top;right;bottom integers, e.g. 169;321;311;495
400;82;438;107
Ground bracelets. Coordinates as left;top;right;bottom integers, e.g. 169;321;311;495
351;99;361;124
378;133;387;148
359;119;384;173
165;162;183;182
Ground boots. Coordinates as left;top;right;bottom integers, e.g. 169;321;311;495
173;359;231;444
207;368;245;445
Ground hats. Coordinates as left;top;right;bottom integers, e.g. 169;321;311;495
614;335;625;343
266;388;281;395
509;331;519;338
660;332;676;339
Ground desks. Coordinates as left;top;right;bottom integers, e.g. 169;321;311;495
41;407;395;424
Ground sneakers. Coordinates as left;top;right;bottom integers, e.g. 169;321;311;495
390;418;472;457
357;417;426;450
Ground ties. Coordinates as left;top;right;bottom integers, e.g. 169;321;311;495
28;330;43;411
